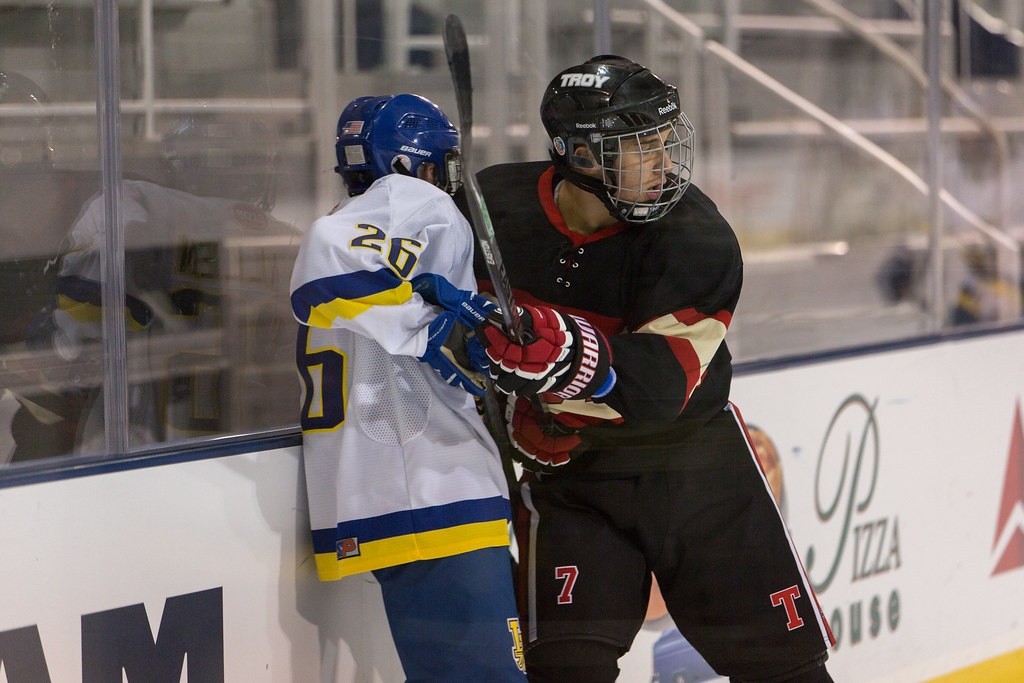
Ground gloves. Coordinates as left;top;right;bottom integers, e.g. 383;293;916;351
466;294;615;406
491;383;627;475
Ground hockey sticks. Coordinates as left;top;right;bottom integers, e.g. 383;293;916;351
432;8;555;433
479;372;525;516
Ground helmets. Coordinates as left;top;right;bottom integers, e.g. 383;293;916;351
541;56;694;226
335;92;464;200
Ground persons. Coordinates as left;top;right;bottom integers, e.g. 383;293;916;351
953;243;1022;328
453;55;839;683
1;69;306;463
288;94;530;683
